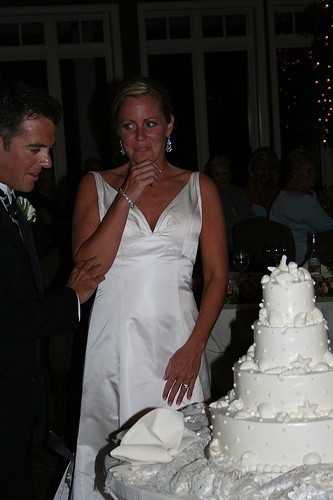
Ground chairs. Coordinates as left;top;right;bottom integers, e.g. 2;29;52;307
232;217;297;270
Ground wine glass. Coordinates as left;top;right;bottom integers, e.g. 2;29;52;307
233;250;250;278
264;241;288;267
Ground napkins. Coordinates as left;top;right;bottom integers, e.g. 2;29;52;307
108;407;202;479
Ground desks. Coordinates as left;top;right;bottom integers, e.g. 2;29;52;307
204;272;333;398
103;401;212;500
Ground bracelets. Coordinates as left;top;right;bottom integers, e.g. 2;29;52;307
119;187;134;208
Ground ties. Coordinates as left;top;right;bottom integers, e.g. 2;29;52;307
10;196;43;295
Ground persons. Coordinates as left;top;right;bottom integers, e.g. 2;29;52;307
53;77;230;500
0;82;106;500
205;146;333;266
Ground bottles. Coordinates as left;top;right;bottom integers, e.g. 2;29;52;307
308;233;321;275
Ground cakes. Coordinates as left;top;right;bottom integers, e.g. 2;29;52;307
208;255;333;473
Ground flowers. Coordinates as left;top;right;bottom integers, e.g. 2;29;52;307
16;195;38;234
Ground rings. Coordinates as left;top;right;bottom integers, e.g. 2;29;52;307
184;384;188;388
